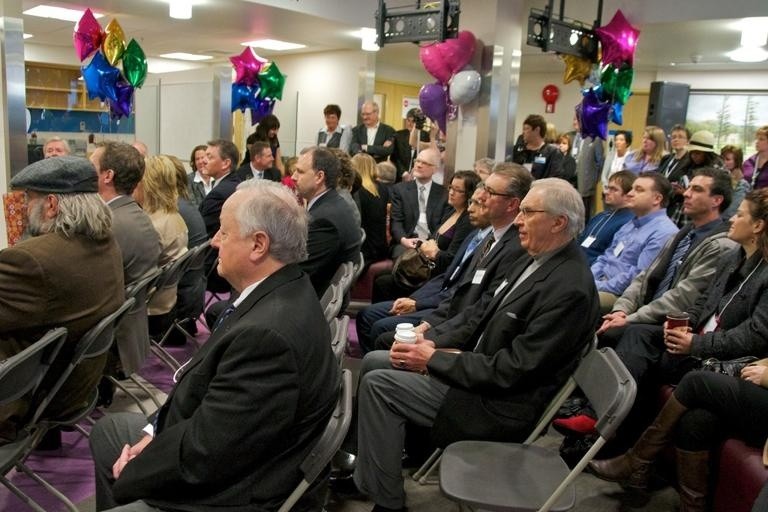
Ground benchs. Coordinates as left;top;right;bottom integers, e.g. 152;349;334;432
657;382;767;512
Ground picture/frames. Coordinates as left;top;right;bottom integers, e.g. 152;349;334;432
373;91;386;123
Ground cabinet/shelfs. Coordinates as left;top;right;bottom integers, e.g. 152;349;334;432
25;61;110;112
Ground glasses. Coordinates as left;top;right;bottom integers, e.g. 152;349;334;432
413;158;435;166
447;184;464;192
467;198;482;208
480;183;515;198
521;207;550;216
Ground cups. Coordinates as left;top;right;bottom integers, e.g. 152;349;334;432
666;311;690;344
394;330;418;352
395;323;416;335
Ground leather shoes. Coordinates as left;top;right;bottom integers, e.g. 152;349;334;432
329;469;369;500
553;416;598;437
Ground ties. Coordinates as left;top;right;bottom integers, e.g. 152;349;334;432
479;236;495;264
418;185;426;212
257;173;263;178
467;234;482;251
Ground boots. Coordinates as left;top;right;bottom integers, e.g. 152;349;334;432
588;391;711;512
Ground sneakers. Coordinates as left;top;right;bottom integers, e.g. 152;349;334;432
32;432;62;456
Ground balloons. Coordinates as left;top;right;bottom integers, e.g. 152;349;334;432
71;10;146;122
564;10;640;135
418;31;483;133
228;46;285;125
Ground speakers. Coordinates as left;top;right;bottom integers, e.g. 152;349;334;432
646;81;690;141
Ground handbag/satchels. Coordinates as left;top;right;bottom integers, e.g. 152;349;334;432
377;162;397;184
702;355;759;377
393;240;437;291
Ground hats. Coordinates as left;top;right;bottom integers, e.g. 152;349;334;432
9;156;99;194
684;131;715;152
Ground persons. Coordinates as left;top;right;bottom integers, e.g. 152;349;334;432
1;155;122;418
86;102;768;510
41;135;70;159
